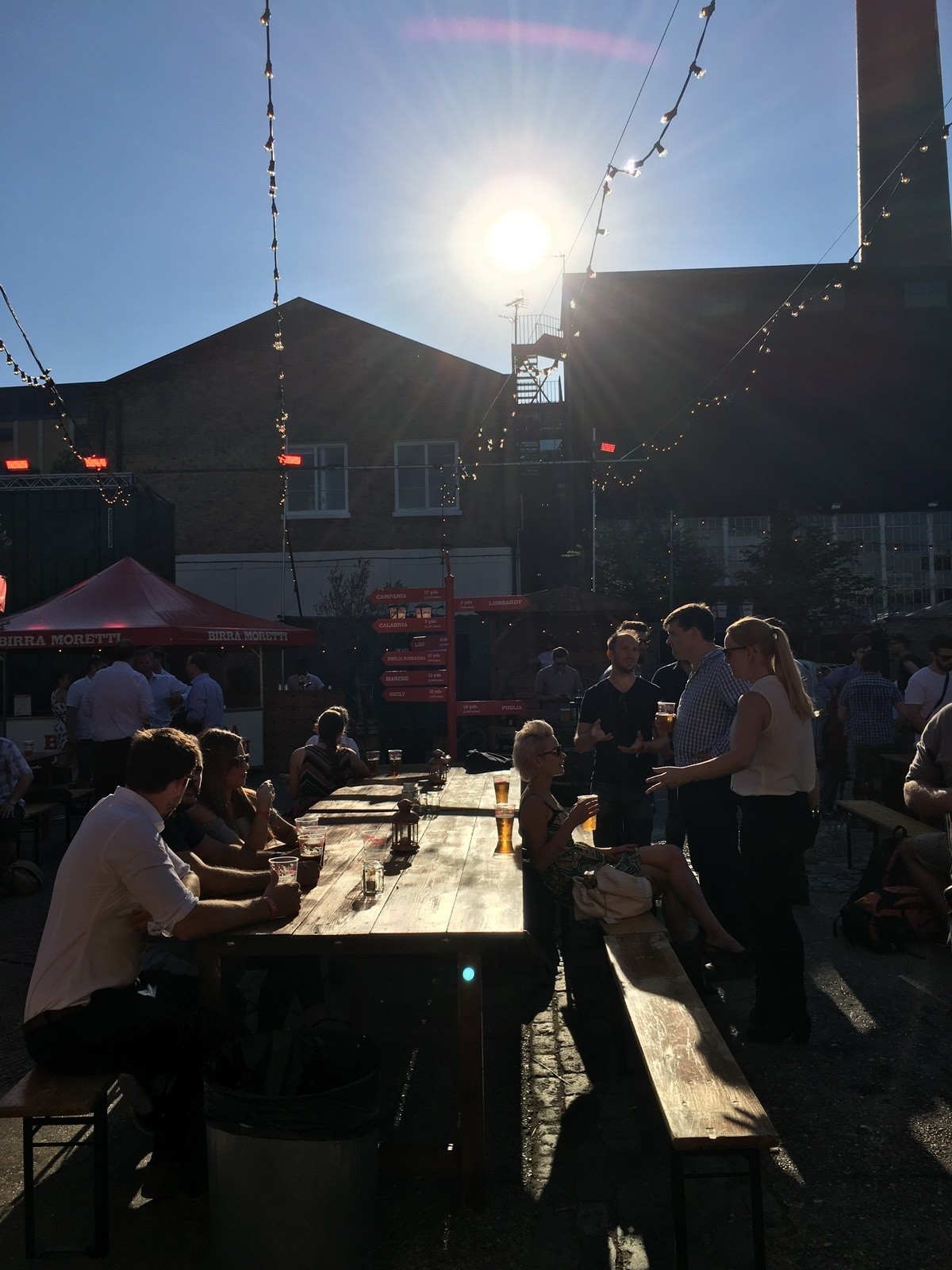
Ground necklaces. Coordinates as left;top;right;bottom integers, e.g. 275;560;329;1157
750;673;776;688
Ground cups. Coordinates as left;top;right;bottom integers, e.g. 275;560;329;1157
658;701;675;722
295;818;319;839
388;750;402;774
23;740;34;757
268;857;299;886
302;826;327;866
366;751;380;775
493;775;510;804
495;803;515;841
577;795;598;831
298;834;323;865
492;841;516;860
362;830;389;865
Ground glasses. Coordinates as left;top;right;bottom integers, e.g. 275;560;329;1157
138;660;152;665
620;695;629;716
536;744;561;757
722;645;756;659
195;765;202;775
555;663;565;666
184;663;194;668
932;650;952;661
888;641;900;647
639;639;651;646
230;752;250;766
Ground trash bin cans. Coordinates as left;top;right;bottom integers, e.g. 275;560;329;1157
204;1030;383;1270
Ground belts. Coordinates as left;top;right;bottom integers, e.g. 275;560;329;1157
23;1005;83;1037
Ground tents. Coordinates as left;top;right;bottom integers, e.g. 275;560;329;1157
0;557;315;709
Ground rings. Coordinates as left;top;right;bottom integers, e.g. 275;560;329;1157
660;783;664;787
587;815;590;818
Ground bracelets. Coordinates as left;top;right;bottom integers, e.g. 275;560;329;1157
258;811;270;818
6;799;18;806
635;743;648;757
263;896;276;921
549;838;564;853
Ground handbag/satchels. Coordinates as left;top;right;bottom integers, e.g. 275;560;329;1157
572;863;652;921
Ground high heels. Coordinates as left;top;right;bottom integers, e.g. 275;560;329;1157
747;1006;812;1045
705;942;756;971
696;976;718;995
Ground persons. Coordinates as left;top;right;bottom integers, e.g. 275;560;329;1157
24;728;322;1198
513;719;754;962
820;631;952;821
901;702;952;940
51;639;226;805
0;737;36;870
574;601;753;955
289;658;326;690
533;647;583;723
287;706;370;817
646;614;818;1044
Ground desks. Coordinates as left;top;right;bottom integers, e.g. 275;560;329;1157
142;757;533;1246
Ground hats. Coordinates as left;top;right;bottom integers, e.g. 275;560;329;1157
552;647;569;663
883;633;908;642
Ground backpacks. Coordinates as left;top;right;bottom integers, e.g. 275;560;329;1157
838;826;943;955
0;859;46;893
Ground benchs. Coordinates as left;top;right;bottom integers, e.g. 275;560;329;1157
565;806;779;1270
1;1049;133;1256
837;799;944;870
15;788;96;868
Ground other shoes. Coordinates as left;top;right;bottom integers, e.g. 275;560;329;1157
117;1074;161;1135
937;938;951;947
704;962;736;981
141;1163;210;1224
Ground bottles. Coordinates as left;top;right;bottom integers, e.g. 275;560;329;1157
425;791;441;817
402;782;419;803
361;860;385;895
